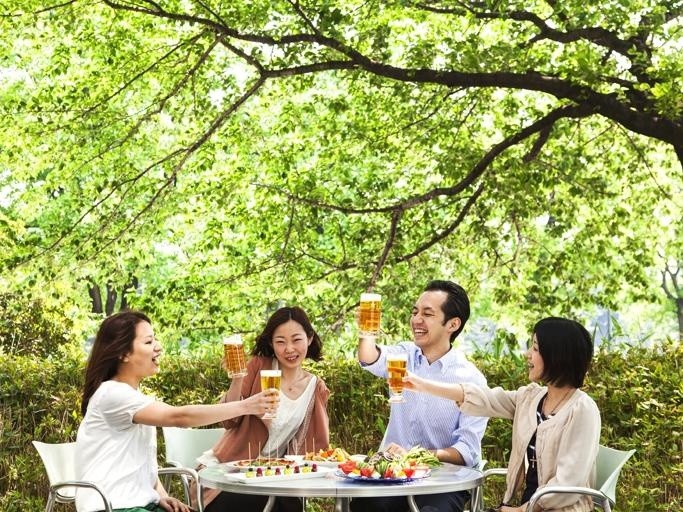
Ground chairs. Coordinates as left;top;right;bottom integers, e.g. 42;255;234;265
342;454;488;512
32;440;205;512
476;444;637;512
162;425;308;512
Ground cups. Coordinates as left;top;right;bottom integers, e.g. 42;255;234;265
257;368;283;422
357;292;382;340
386;357;407;403
221;335;248;380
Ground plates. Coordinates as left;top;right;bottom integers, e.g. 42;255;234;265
226;456;307;470
222;470;328;484
283;451;343;468
335;468;431;483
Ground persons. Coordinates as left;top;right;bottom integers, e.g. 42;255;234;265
384;316;602;512
72;311;280;512
348;279;489;512
186;307;332;512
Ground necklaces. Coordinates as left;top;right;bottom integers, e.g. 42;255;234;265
280;367;301;392
539;386;571;414
